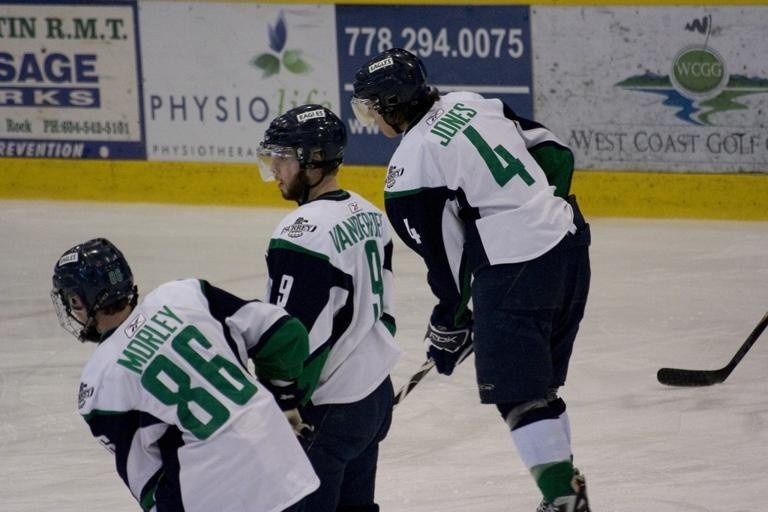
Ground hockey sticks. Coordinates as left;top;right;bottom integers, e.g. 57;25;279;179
658;314;768;387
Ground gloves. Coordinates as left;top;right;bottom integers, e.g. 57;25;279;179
422;303;475;375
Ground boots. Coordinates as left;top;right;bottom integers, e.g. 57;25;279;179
536;469;593;511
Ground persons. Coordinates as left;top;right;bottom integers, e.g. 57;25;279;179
349;47;593;512
255;104;398;512
51;238;326;512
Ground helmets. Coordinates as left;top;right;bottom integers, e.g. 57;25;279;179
51;236;135;306
263;101;348;164
350;46;432;115
